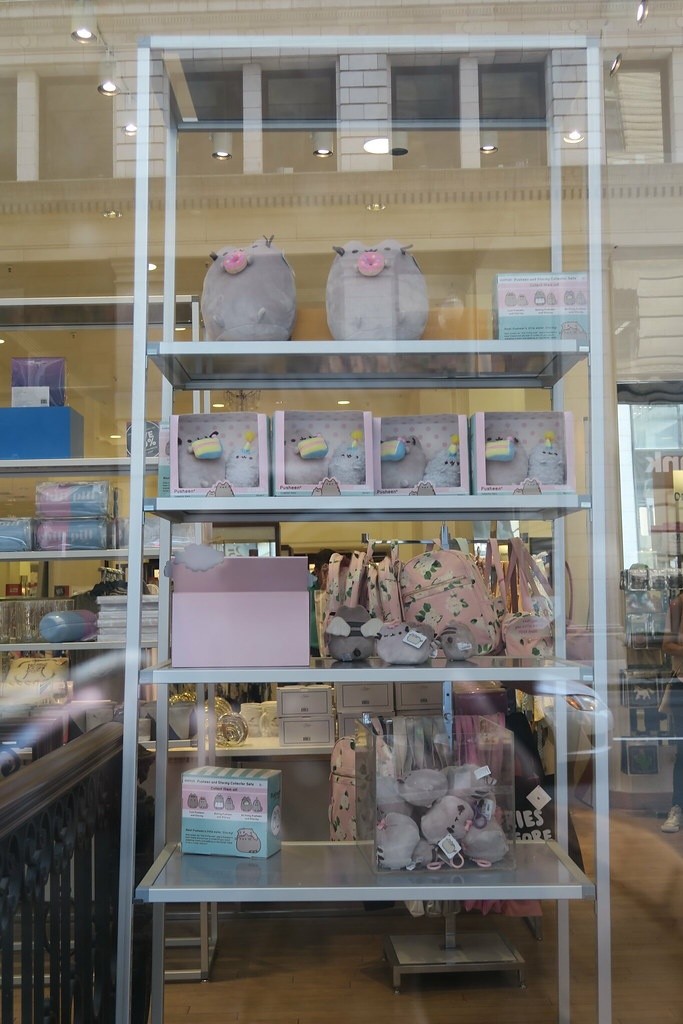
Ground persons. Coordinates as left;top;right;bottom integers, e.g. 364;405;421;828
661;593;683;832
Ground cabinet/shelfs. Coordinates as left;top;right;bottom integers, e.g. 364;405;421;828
116;28;611;1024
0;295;218;987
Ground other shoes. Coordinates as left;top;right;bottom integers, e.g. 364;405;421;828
661;805;682;832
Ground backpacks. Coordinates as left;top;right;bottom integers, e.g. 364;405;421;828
398;539;500;656
323;553;404;655
329;731;412;840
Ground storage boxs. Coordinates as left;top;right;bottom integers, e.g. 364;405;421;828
354;716;516;876
337;712;395;740
169;412;269;498
395;681;453;711
397;710;443;717
492;271;590;346
0;407;84;460
11;357;65;407
335;682;394;713
156;421;170;498
272;411;374;495
470;410;575;495
181;765;282;859
373;412;470;497
127;421;160;458
280;714;335;747
35;481;212;549
0;517;40;552
277;685;332;718
171;557;310;668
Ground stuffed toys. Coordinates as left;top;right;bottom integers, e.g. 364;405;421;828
192;233;562;872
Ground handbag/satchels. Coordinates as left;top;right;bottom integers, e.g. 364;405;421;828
658;678;672;714
487;536;562;657
498;713;584;875
